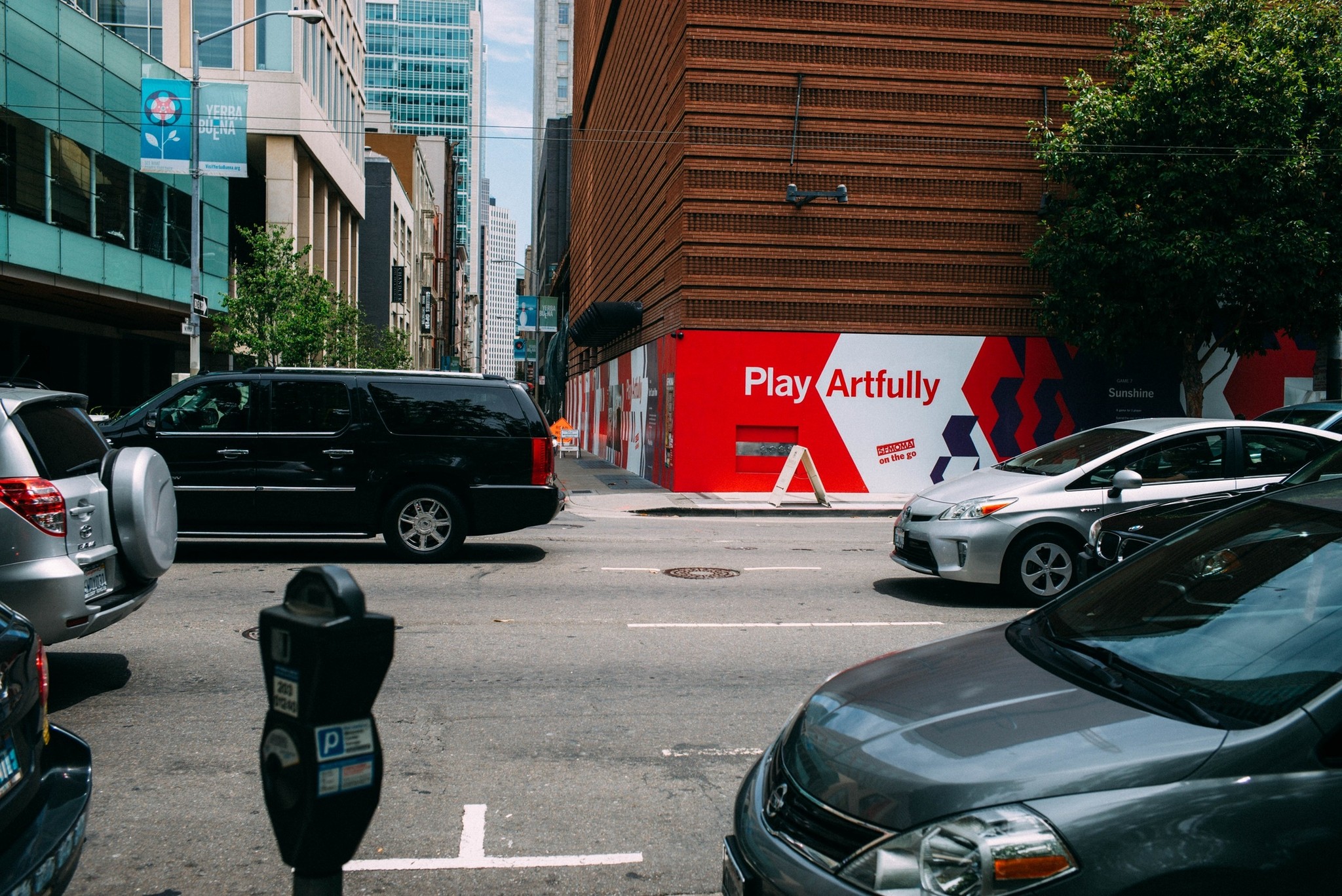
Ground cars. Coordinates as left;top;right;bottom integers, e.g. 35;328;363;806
1205;399;1342;465
888;416;1342;610
717;474;1342;896
0;600;95;896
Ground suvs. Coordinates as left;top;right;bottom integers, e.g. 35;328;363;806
59;364;568;564
0;377;179;648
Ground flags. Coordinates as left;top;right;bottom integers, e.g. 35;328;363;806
518;296;537;331
539;297;558;332
140;78;193;174
514;340;536;361
198;81;250;177
441;355;449;372
450;356;460;373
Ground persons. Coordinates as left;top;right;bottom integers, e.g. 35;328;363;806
1124;440;1204;483
214;386;246;432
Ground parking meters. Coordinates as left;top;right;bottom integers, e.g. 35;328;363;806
257;565;397;896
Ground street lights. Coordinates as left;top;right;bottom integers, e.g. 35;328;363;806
184;6;325;380
496;316;527;382
461;356;481;372
492;258;540;405
449;340;474;373
